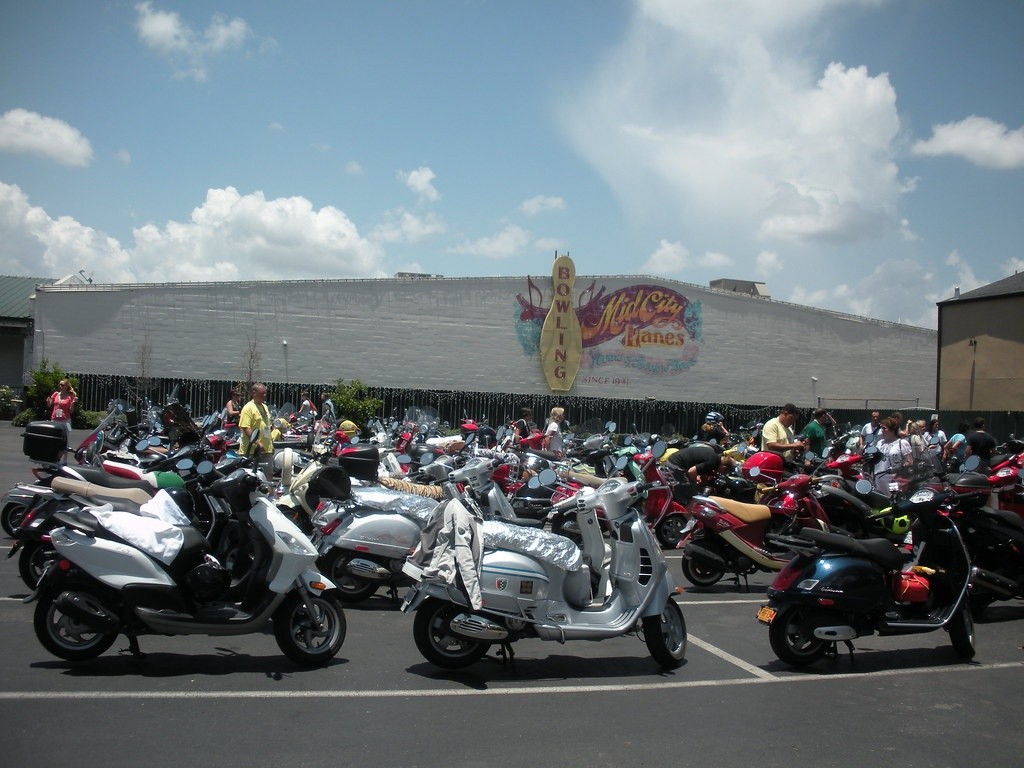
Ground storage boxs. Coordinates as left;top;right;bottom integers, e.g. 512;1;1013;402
20;420;68;464
742;451;786;482
339;443;380;481
1007;440;1024;454
479;426;498;448
946;474;993;508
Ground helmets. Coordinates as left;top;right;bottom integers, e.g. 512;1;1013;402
165;486;196;520
187;560;232;602
880;506;910;534
308;466;351;501
705;411;724;424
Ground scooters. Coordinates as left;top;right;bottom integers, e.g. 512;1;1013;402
0;399;1024;625
21;443;347;670
758;472;998;669
404;437;692;669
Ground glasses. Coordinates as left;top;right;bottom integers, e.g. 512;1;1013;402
881;426;888;429
59;384;65;386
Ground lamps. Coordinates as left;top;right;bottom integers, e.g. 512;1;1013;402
968;336;977;346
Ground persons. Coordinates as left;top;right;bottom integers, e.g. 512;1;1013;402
965;417;998;467
799;408;828;456
761;403;805;462
666;445;735;502
320;393;336;422
226;390;241;435
543;407;569;452
298;391;312;425
46;379;77;465
700;411;729;444
237;383;274;454
514;408;531;447
859;410;968;497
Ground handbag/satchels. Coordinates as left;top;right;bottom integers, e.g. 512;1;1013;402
44;392;58;421
221;408;231;423
